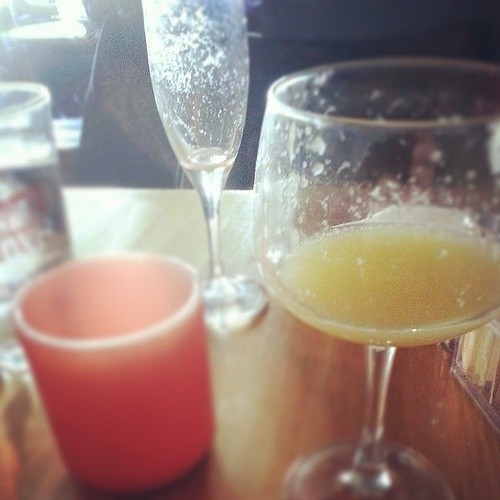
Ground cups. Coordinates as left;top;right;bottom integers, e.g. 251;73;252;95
0;83;78;375
13;250;214;490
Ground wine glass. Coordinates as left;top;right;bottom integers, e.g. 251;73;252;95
250;57;498;498
143;2;266;334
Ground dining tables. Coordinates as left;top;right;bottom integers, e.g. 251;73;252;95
0;186;500;500
7;19;91;118
